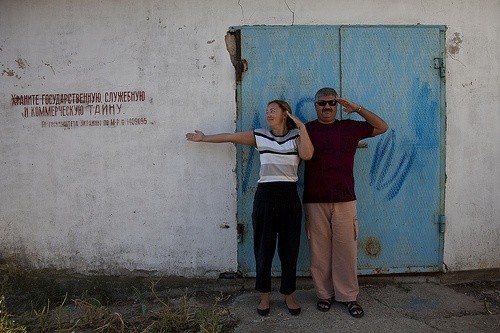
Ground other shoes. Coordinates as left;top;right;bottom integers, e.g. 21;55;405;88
257;298;270;316
284;297;301;316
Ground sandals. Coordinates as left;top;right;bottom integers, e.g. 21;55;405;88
345;301;364;318
317;296;333;312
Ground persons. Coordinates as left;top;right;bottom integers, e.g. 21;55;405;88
297;88;389;318
185;100;314;317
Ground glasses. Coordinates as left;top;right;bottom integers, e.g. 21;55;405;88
315;99;337;106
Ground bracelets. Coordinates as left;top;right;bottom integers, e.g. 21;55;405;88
357;105;362;113
